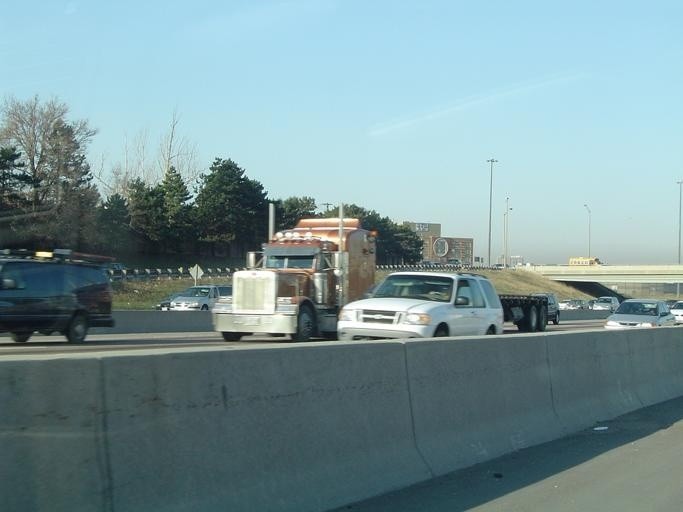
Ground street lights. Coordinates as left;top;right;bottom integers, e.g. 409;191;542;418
584;204;591;256
503;195;513;266
676;181;683;264
486;158;497;267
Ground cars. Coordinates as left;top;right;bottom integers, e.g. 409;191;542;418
417;257;509;270
155;291;182;311
531;292;682;330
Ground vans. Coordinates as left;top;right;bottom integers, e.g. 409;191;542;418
170;285;232;311
1;245;116;348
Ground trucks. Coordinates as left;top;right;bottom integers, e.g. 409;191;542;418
210;202;550;341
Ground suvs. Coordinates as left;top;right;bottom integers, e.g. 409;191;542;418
335;270;505;343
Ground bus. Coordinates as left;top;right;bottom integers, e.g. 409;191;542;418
568;257;602;264
